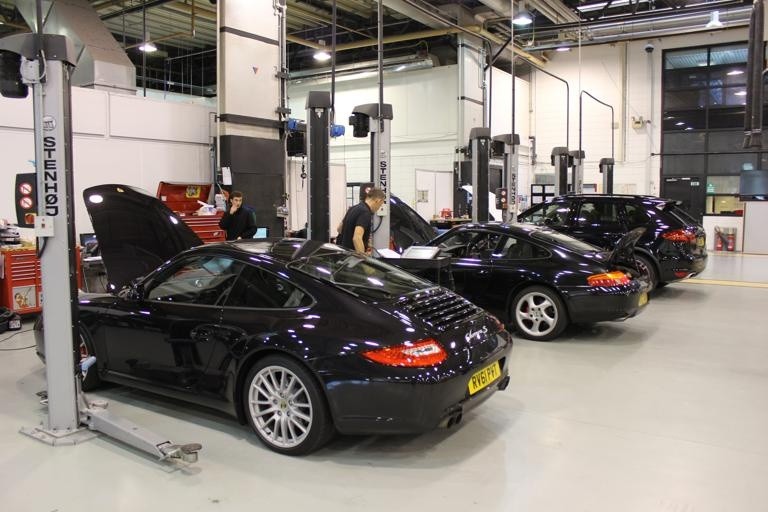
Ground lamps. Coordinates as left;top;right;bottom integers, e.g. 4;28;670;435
139;11;159;55
705;9;724;31
513;1;534;25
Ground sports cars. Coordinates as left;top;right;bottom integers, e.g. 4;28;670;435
27;185;513;456
360;187;648;341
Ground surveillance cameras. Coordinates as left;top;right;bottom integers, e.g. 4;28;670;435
644;43;654;53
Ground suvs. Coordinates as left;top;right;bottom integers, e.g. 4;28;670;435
464;181;708;296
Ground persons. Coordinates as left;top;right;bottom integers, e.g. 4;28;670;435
295;223;308;239
336;188;385;253
219;191;257;241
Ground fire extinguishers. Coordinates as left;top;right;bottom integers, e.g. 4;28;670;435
714;225;735;251
